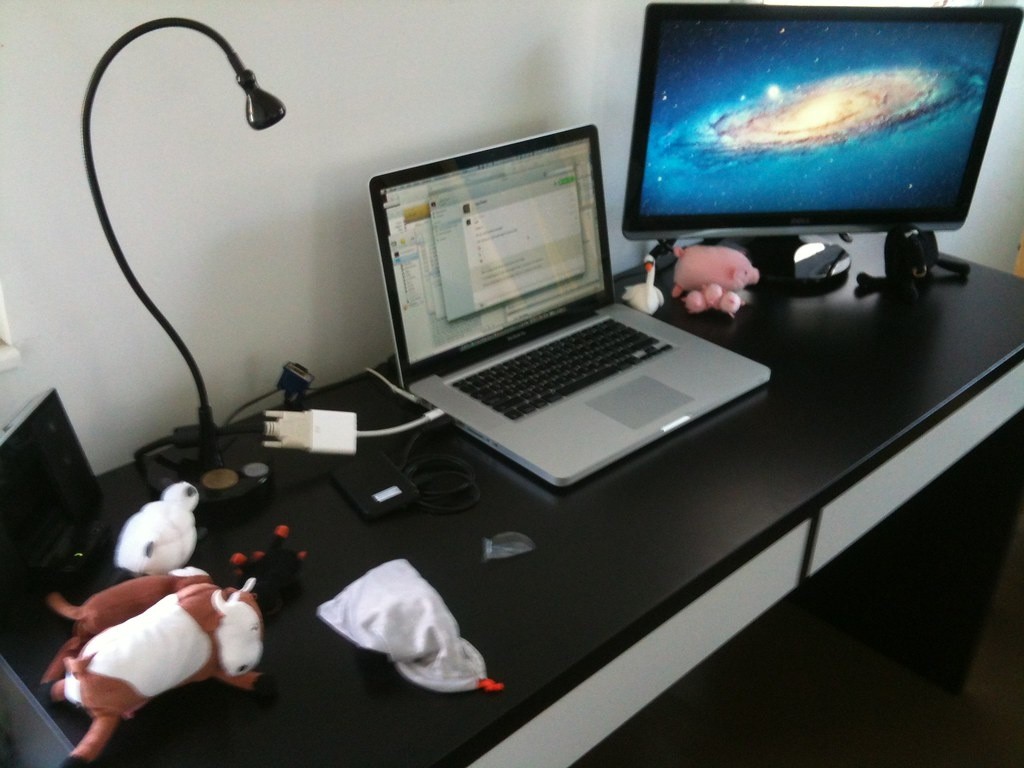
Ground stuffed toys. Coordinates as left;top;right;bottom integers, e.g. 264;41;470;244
42;567;282;768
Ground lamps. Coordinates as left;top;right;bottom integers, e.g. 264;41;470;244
80;17;288;508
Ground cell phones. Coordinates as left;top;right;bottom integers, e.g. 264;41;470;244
329;449;421;522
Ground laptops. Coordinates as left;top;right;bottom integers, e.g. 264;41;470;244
365;122;771;485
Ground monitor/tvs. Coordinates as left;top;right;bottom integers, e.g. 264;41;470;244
620;3;1022;288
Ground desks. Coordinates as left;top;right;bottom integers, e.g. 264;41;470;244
0;248;1024;768
